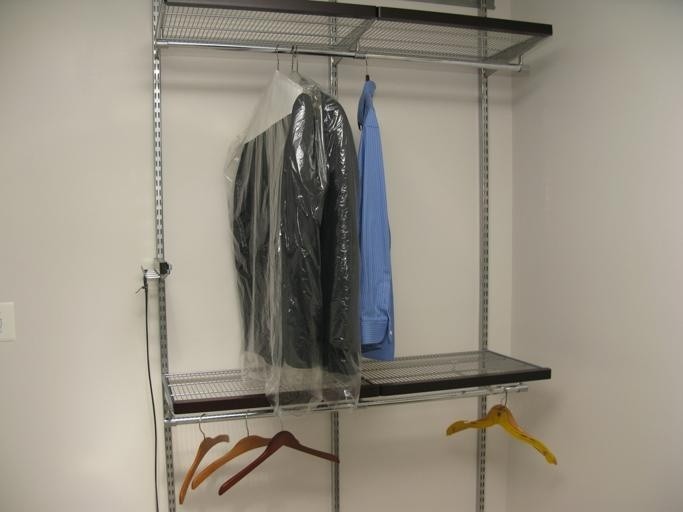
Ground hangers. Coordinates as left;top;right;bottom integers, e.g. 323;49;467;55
445;386;556;465
270;40;369;89
177;406;340;505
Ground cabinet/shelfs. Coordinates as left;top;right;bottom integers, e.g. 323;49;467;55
149;0;552;512
